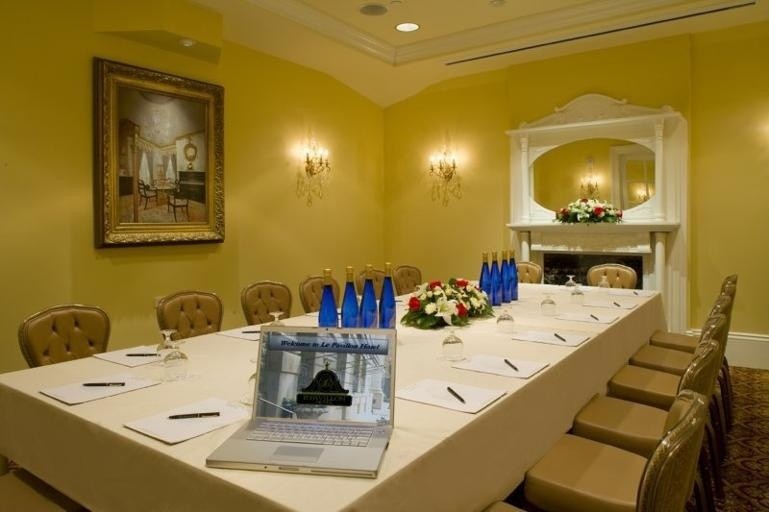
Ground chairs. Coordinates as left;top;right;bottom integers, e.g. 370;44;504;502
394;263;424;294
586;262;638;290
236;280;294;324
484;274;741;511
297;278;343;312
154;284;223;342
19;303;112;369
515;261;543;283
355;262;390;301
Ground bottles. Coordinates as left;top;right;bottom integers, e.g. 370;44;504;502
380;263;397;329
319;268;339;330
360;264;380;328
340;265;360;328
479;248;518;308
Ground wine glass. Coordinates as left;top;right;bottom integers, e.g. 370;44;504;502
597;275;611;294
540;291;558;318
268;311;285;326
247;358;256;402
494;306;516;334
564;274;585;307
440;324;466;361
155;328;190;380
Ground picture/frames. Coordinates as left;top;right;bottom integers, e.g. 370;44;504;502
94;53;227;251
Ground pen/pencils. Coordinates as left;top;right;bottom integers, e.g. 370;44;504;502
503;357;520;373
554;332;566;342
167;412;221;420
126;353;161;357
613;301;620;307
242;330;261;334
633;291;639;296
395;299;403;303
446;386;467;405
590;314;600;320
337;312;341;315
82;382;126;387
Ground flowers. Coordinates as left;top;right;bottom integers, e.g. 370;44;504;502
400;278;495;332
552;199;625;226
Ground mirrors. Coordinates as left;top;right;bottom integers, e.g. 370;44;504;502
523;135;666;218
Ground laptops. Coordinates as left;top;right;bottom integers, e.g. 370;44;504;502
206;325;397;479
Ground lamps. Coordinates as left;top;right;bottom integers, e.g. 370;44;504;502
629;174;656;203
285;121;345;206
575;154;603;200
418;125;474;211
